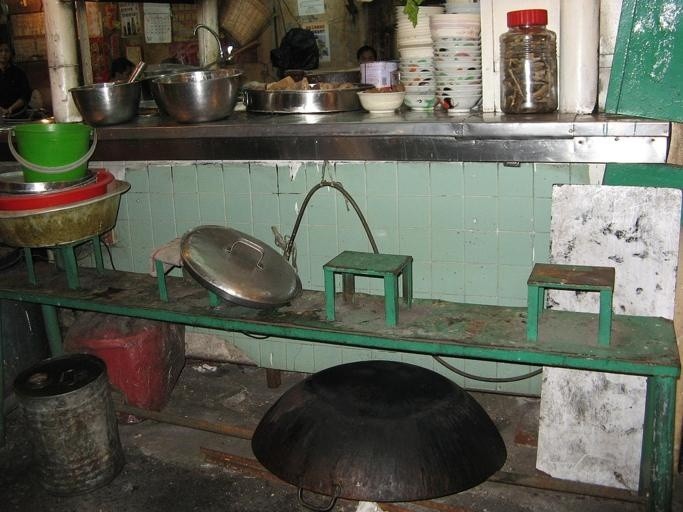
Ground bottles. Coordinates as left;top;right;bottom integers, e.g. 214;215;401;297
501;10;556;113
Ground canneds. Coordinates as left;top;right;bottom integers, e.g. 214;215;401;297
361;61;398;88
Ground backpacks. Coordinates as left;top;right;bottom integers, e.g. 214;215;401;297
270;28;320;69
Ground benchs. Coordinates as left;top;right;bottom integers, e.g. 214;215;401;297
2;260;683;512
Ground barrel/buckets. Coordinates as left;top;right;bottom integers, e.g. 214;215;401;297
9;350;124;497
8;122;98;183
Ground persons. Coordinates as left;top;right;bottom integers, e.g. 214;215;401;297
357;46;377;69
108;57;136;83
0;40;32;117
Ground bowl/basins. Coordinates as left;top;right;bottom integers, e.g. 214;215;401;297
430;12;482;117
151;68;243;125
68;81;143;126
0;167;131;250
356;91;406;115
396;5;445;113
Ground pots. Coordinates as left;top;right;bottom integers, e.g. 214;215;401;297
181;224;301;309
251;359;508;508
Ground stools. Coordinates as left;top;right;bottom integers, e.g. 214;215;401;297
23;234;105;292
526;263;616;349
321;250;413;330
154;237;220;307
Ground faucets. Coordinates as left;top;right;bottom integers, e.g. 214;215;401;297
193;24;235;68
